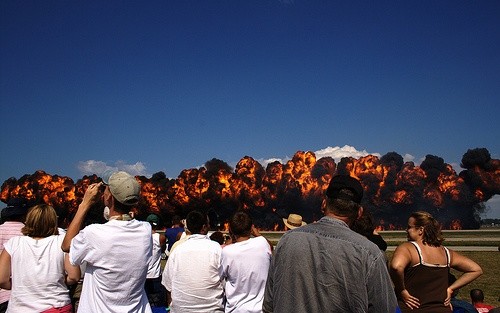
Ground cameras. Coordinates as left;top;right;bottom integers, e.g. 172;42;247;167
101;185;106;194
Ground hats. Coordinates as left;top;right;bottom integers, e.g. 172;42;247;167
146;214;159;224
326;175;363;204
102;168;139;206
283;213;307;230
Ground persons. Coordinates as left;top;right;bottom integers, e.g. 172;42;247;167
160;211;272;313
0;204;82;313
61;172;166;313
390;211;500;313
261;175;398;313
